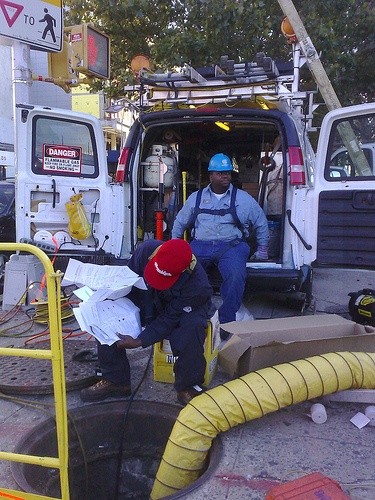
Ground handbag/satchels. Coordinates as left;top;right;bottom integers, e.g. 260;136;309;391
65;192;91;241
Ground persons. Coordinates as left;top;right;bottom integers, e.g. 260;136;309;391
80;239;216;406
171;153;270;341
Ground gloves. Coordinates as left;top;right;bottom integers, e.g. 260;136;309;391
250;246;269;260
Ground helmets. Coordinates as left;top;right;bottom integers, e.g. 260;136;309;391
208;153;233;171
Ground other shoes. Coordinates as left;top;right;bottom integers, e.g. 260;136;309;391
220;328;230;340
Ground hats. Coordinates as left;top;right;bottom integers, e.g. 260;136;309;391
144;239;192;291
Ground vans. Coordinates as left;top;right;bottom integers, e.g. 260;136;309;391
15;91;375;314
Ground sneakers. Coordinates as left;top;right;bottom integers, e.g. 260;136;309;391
177;385;208;407
80;379;131;402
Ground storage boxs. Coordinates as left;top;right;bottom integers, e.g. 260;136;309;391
242;182;260;202
153;309;222;387
219;312;375;377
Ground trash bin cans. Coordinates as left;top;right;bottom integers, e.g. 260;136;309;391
0;181;16;242
